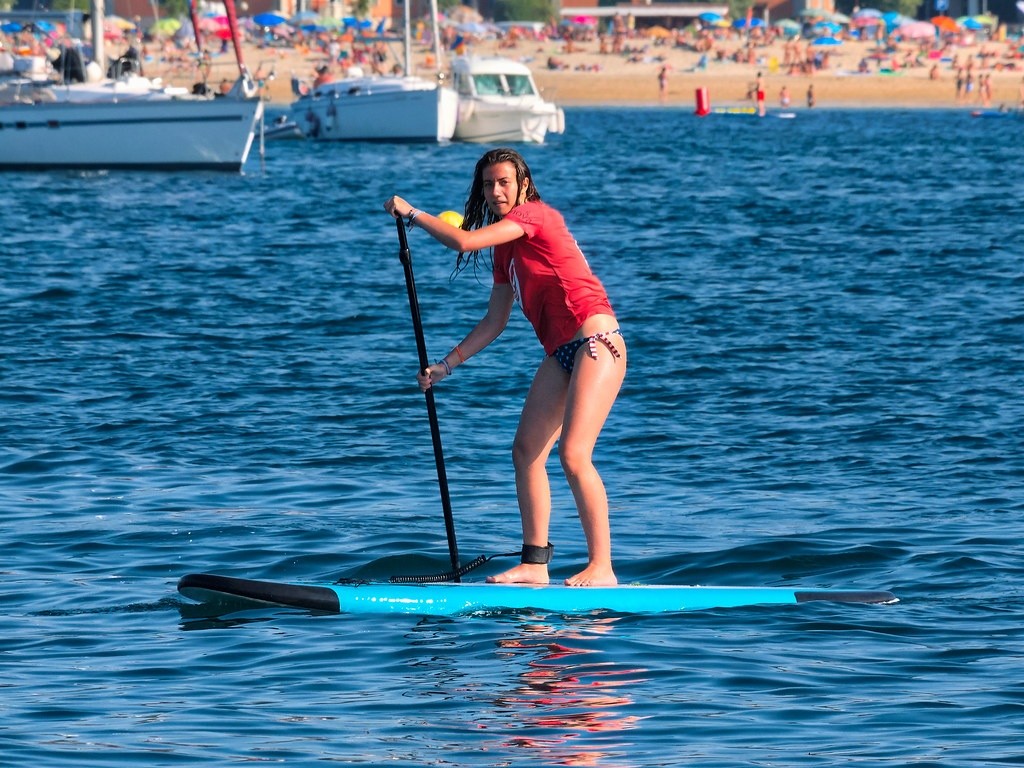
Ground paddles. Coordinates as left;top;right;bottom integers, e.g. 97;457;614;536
394;212;462;583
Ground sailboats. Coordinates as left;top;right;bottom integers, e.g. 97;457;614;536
291;1;459;143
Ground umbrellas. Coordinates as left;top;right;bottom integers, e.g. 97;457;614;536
0;14;243;41
700;8;994;46
427;15;482;33
571;16;597;24
255;11;374;34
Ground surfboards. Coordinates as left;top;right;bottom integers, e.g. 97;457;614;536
177;572;902;615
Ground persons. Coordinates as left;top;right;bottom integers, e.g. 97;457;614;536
0;28;402;95
930;65;937;79
383;148;627;586
780;86;789;106
413;23;534;67
538;13;1024;73
659;66;667;90
956;64;975;92
806;84;815;108
978;75;991;106
746;72;764;100
133;15;145;42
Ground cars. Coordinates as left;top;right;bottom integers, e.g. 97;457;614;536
496;21;543;35
0;11;143;86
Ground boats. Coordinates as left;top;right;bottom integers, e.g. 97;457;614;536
1;1;272;170
450;58;565;143
255;121;304;141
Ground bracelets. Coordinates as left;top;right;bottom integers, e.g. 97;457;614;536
406;211;421;232
456;346;464;362
441;359;451;375
